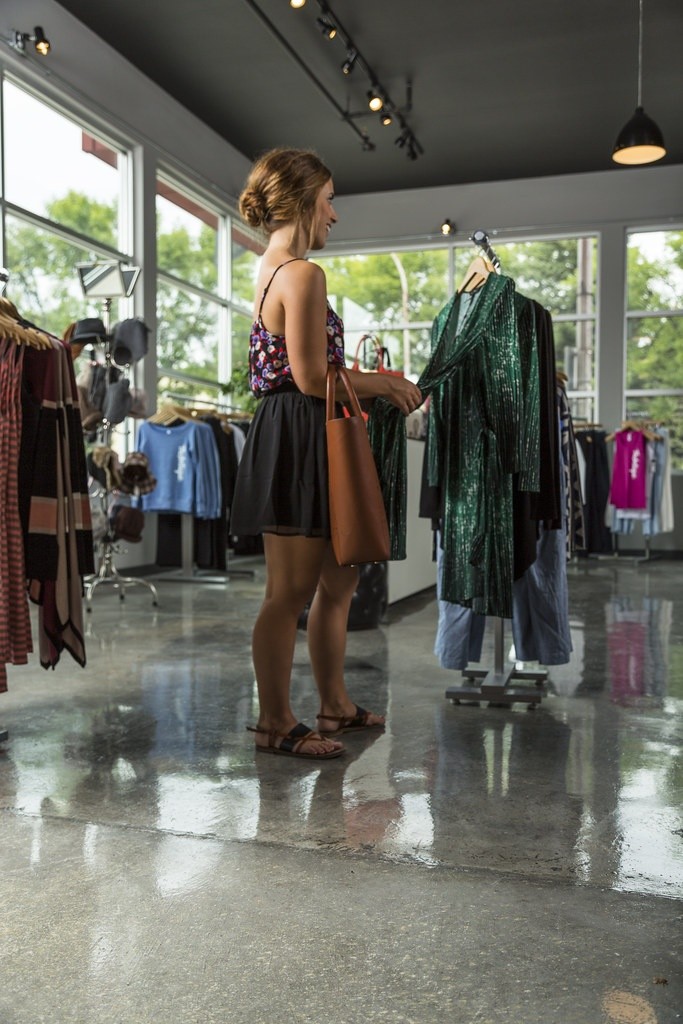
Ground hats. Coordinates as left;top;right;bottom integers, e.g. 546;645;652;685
61;318;157;559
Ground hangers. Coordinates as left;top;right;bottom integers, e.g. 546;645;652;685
147;392;253;435
457;235;502;294
604;411;663;443
0;267;52;351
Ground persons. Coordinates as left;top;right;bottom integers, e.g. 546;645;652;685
231;147;422;757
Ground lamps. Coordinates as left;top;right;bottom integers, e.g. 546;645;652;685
12;26;51;56
315;18;418;161
611;0;668;166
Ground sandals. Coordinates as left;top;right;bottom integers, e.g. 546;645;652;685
317;703;384;737
247;721;346;759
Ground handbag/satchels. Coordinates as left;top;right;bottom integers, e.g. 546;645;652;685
326;363;392;568
340;336;404;421
